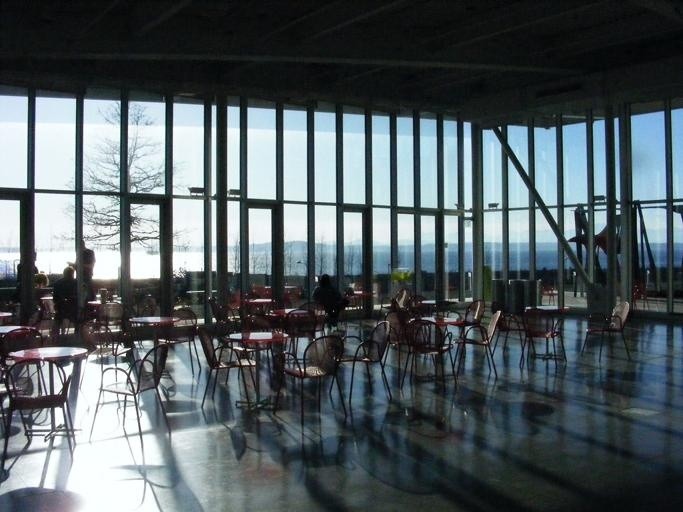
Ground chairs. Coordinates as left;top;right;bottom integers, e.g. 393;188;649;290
382;309;419;375
0;275;650;346
195;326;259;416
452;311;501;381
582;300;632;363
328;321;393;406
399;319;457;393
0;383;22;428
520;308;568;370
0;358;76;469
96;325;131;370
89;344;171;448
0;327;43;366
272;335;348;426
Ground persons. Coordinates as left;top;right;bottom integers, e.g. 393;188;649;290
11;241;95;340
312;273;344;328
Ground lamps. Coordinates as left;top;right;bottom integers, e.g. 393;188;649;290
455;191;608;208
186;181;244;201
